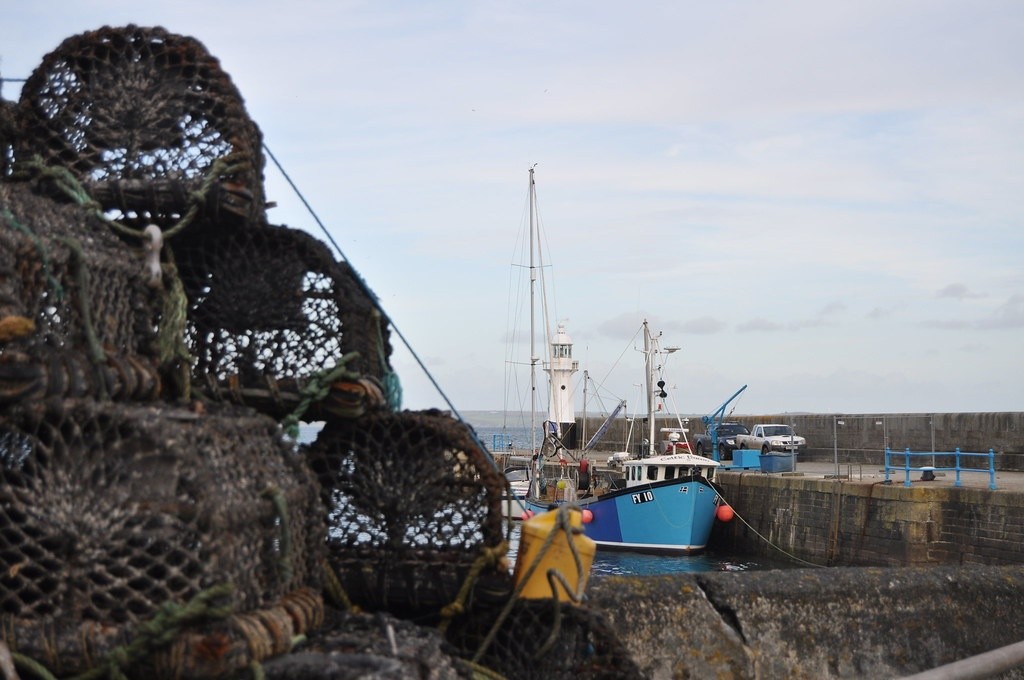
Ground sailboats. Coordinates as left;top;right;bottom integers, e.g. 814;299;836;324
501;157;734;554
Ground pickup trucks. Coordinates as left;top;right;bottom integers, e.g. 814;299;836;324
735;424;808;461
692;422;752;459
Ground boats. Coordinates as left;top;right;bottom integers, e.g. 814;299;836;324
496;467;542;522
